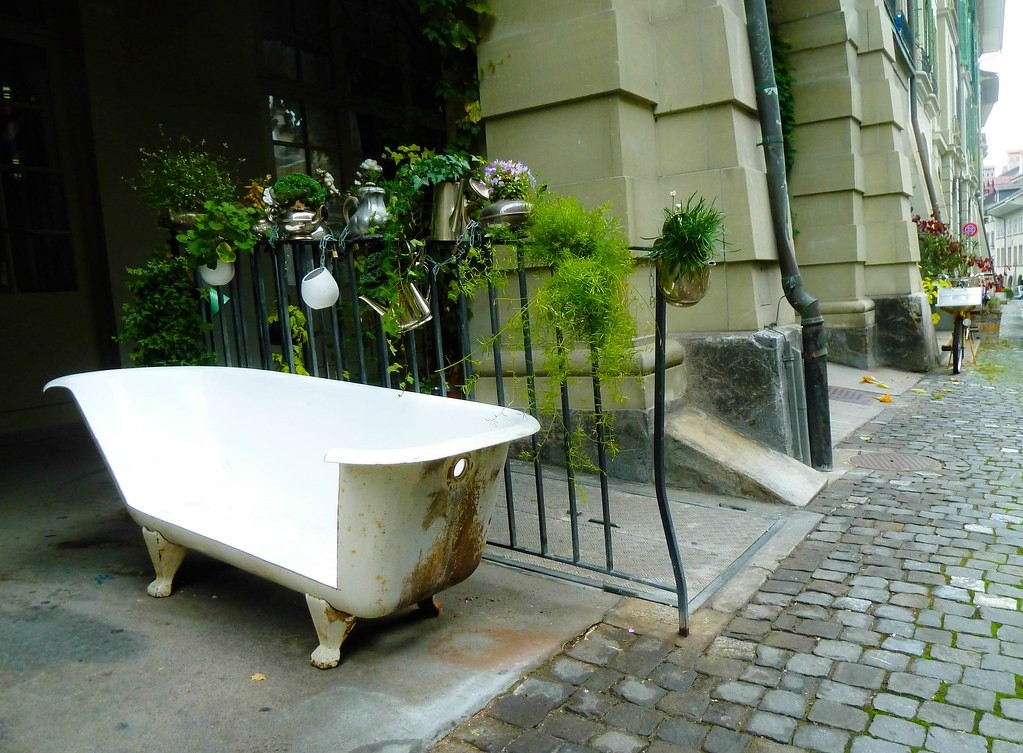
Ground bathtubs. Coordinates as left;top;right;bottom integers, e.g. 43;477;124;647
43;365;545;669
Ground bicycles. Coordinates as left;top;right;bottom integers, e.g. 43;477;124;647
933;271;992;374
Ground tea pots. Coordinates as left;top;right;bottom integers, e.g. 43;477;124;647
342;181;388;236
357;265;433;336
432;176;469;242
275;203;332;243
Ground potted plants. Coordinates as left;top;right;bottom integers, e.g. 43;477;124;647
119;114;739;337
